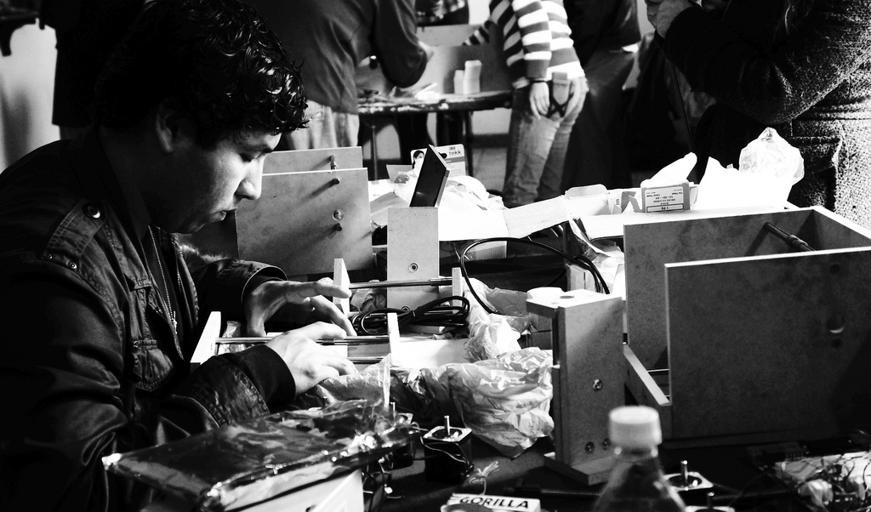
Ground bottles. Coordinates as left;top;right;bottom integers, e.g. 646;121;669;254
593;404;686;512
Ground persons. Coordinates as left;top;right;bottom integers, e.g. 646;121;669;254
0;0;358;512
656;0;871;227
269;0;435;149
459;0;588;207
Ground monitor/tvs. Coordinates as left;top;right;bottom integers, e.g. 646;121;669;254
408;144;450;207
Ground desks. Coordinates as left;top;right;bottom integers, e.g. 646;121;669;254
357;91;510;179
